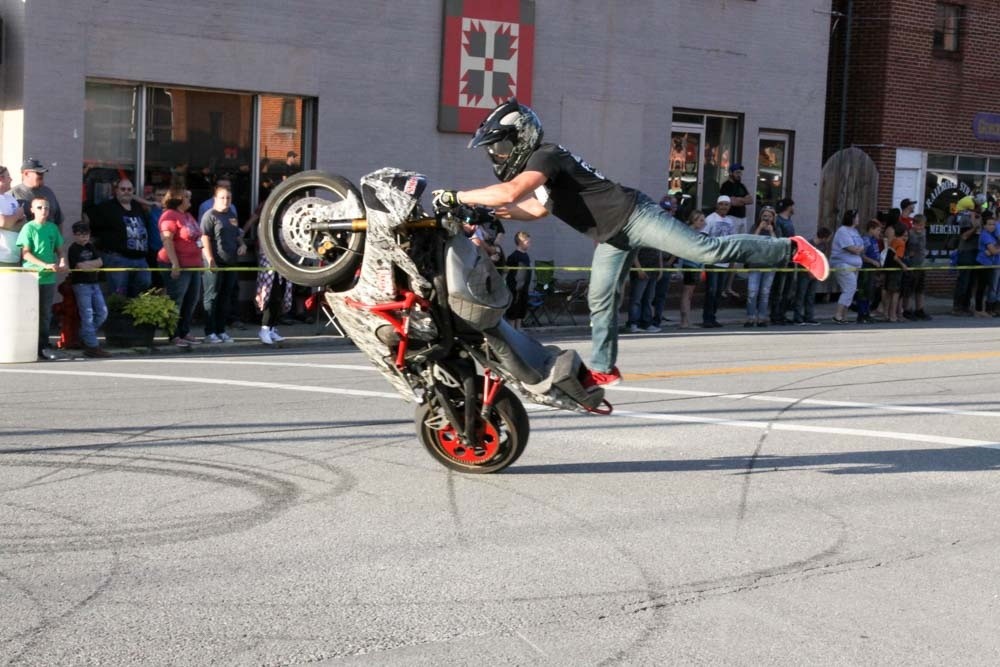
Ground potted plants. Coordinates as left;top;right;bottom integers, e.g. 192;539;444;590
105;287;180;349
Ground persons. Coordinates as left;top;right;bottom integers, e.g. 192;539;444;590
721;162;748;233
67;221;112;358
505;231;530;331
83;151;319;348
432;99;829;393
11;154;64;249
15;196;67;360
624;187;1000;332
470;221;506;276
0;165;27;266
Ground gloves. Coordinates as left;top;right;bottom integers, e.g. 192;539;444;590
431;189;461;216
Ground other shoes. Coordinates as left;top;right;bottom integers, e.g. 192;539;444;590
702;321;714;329
743;316;823;328
83;346;113;359
857;308;933;323
712;321;723;328
832;316;844;325
39;349;60;361
841;320;850;326
950;306;1000;317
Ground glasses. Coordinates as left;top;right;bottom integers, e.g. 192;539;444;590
118;187;134;192
35;206;49;210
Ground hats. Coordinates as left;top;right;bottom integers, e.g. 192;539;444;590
729;163;745;173
717;195;731;203
72;221;91;234
900;198;917;209
19;158;49;173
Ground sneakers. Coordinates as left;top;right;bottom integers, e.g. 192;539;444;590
216;333;234;343
642;325;662;333
625;324;643;333
789;235;830;282
181;334;202;345
204;333;223;343
269;330;284;342
258;330;273;344
169;337;188;347
580;366;623;394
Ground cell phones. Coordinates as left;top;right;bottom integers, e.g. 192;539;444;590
763;217;769;224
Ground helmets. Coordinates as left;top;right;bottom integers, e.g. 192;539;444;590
466;96;544;183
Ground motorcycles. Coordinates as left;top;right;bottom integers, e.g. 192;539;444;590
256;165;612;479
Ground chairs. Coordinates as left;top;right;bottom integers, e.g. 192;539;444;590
520;261;581;328
